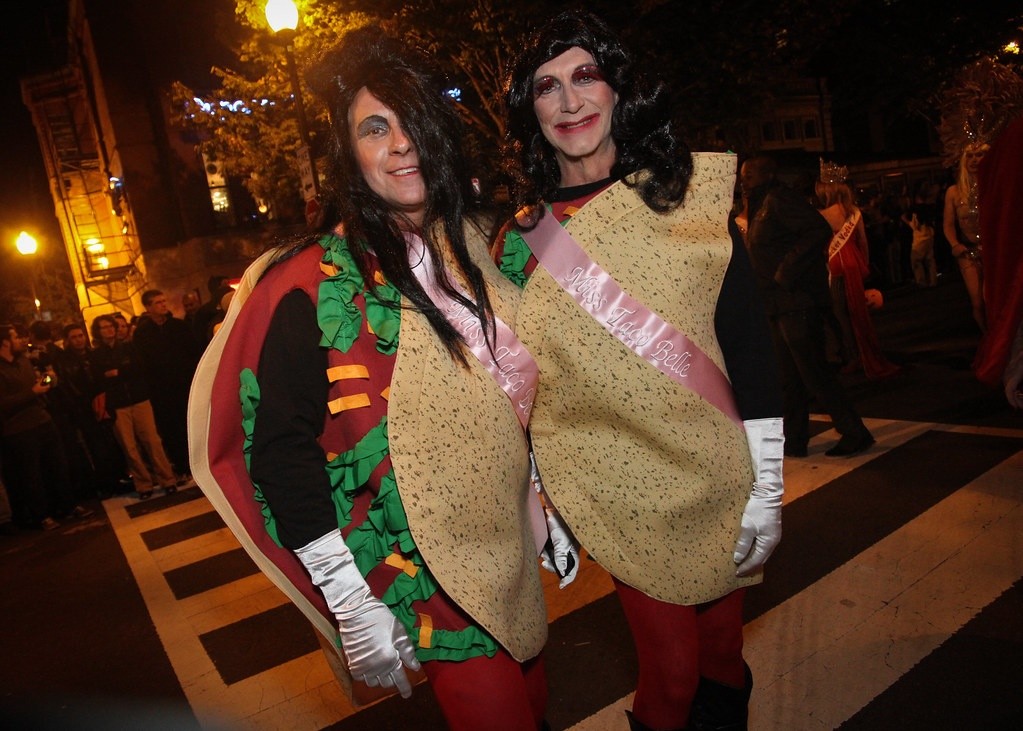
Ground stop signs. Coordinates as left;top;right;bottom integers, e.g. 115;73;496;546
305;199;321;222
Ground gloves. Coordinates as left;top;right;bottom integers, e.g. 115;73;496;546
529;452;579;589
734;416;786;578
294;527;420;698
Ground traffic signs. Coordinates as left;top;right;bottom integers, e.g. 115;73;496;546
296;147;317;202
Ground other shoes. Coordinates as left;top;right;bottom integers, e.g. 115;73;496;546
140;491;151;500
65;506;93;520
785;448;807;457
165;486;176;495
40;516;59;530
825;438;871;457
176;473;192;486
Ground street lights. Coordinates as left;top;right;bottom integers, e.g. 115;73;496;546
13;230;50;331
264;0;323;206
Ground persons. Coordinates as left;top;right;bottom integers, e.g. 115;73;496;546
1;274;235;533
488;9;787;731
728;51;1023;459
186;31;580;730
